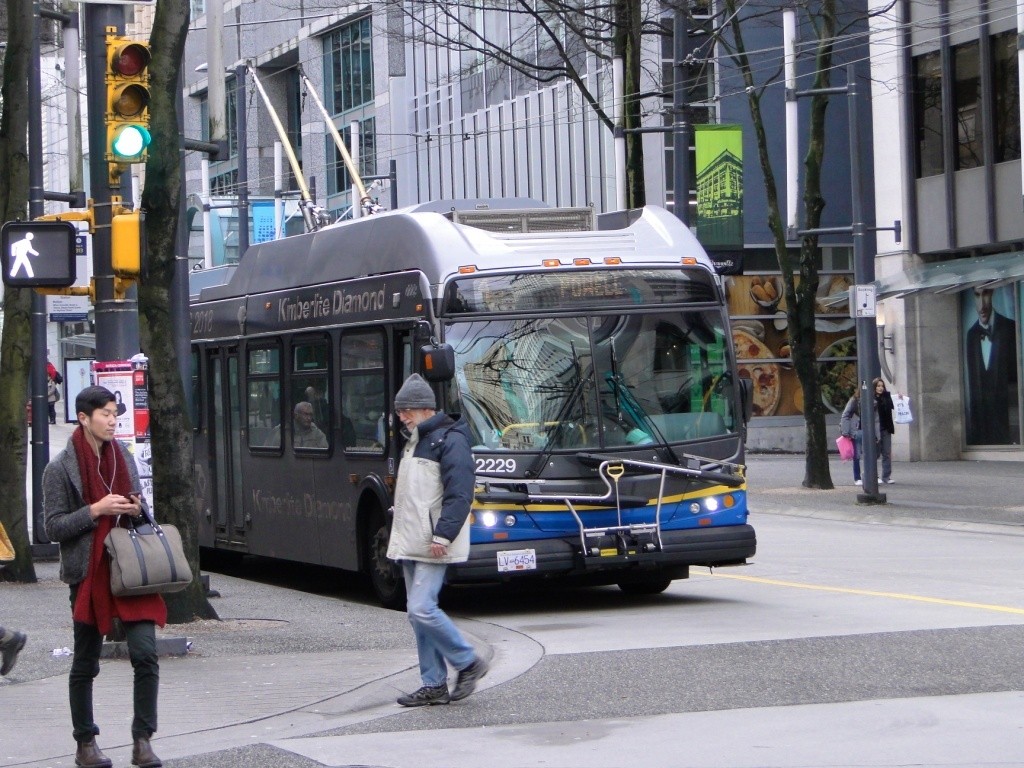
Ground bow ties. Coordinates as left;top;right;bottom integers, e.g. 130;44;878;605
978;325;992;340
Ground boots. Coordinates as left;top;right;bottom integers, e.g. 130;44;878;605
132;734;162;767
74;735;112;768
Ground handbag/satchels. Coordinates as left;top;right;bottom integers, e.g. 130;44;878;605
836;436;855;460
891;394;912;423
104;502;193;596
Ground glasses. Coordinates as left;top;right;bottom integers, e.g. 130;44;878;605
396;409;411;417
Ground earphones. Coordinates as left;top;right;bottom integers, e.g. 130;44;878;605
84;420;87;425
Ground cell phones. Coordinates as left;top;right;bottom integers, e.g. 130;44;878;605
126;491;141;504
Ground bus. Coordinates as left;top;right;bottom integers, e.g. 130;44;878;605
189;57;757;609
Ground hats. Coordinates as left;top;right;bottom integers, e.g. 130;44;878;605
394;373;435;409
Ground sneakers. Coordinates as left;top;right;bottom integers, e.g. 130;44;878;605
451;658;488;701
398;682;449;706
0;630;27;676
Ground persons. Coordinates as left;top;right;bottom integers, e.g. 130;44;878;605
45;348;63;415
0;522;27;675
303;386;357;447
390;372;490;706
46;372;61;425
966;283;1020;444
835;383;881;486
872;376;895;483
43;386;166;768
265;401;329;449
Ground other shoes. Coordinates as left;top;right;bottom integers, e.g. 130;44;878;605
878;479;883;483
885;478;895;483
855;480;862;485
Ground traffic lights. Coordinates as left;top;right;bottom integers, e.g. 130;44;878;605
5;221;82;291
105;39;153;168
111;211;150;283
